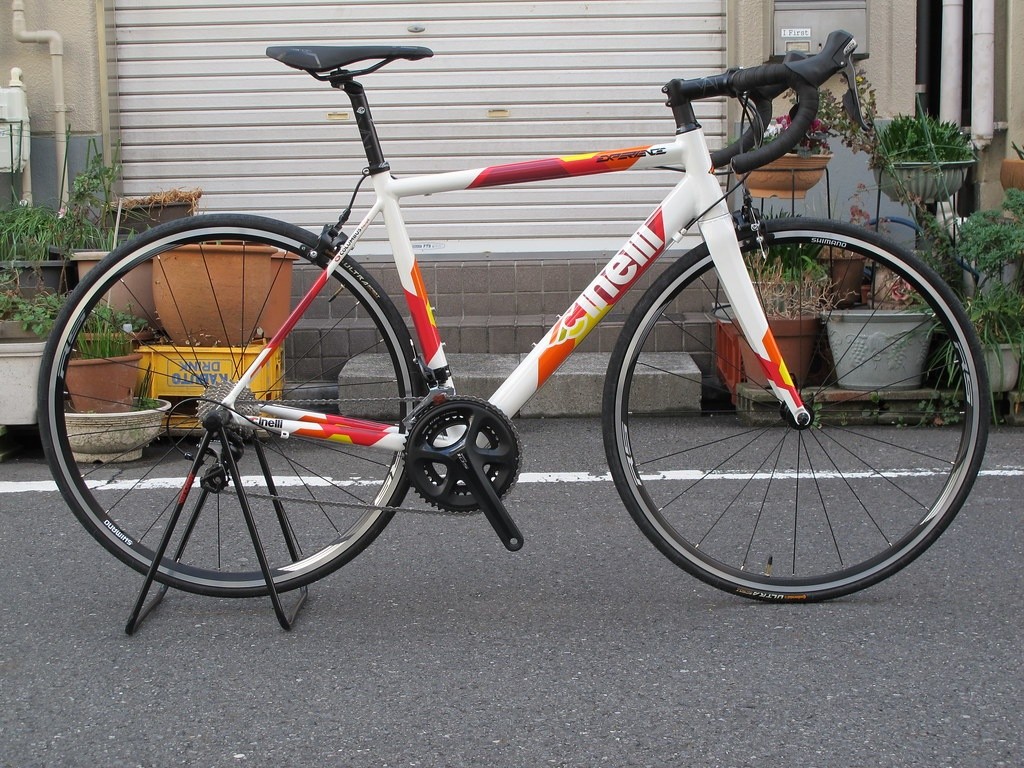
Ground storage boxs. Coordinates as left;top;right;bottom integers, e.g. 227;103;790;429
136;343;285;429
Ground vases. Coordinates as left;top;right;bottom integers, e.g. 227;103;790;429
732;153;832;199
255;252;300;339
149;243;278;348
111;201;193;237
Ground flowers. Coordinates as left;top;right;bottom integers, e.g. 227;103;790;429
725;112;832;160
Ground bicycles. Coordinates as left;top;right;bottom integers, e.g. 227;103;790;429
37;31;994;636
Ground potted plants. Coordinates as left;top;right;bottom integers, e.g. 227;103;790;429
0;119;171;462
728;113;1024;424
